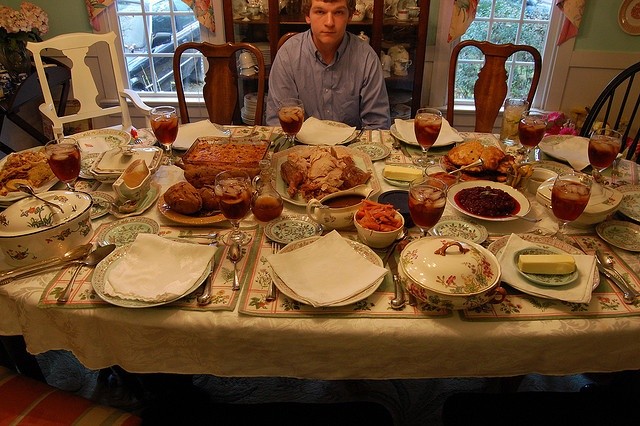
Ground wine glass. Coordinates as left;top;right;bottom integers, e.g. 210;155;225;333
415;107;442;167
213;168;254;246
586;128;625;185
45;136;92;192
550;171;592;240
406;175;448;242
517;115;548;166
148;106;180;162
277;98;306;154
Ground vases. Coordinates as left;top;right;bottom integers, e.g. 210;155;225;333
0;31;43;74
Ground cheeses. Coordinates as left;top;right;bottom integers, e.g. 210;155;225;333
383;166;424;182
518;254;576;276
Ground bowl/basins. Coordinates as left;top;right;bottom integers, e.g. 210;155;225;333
0;190;92;265
185;133;272;178
394;234;506;312
352;208;405;247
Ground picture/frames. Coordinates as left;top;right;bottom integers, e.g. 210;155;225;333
39;100;93;143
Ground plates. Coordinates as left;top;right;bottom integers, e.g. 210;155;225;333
380;161;426;188
83;191;113;220
168;116;230;151
433;216;487;249
445;179;533;224
264;215;323;244
244;41;271;69
537;133;598;166
487;234;600;299
595;219;640;252
69;128;133;154
269;143;381;207
347;140;392;162
389;116;456;149
619;189;640;221
94;238;215;307
294;117;362;147
93;217;161;249
105;182;158;218
267;234;387;305
157;183;228;225
240;91;269;126
0;142;62;204
514;245;578;286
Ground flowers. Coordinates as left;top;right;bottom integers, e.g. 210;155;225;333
0;2;49;39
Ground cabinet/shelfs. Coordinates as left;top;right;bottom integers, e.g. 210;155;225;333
223;0;430;126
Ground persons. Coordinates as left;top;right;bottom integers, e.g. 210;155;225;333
266;1;391;133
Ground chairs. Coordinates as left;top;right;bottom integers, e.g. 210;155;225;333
26;30;157;144
579;61;640;165
447;40;542;134
173;41;265;125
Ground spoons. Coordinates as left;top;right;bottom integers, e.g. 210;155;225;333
228;239;244;291
0;241;116;286
595;251;640;298
1;243;92;283
17;183;63;214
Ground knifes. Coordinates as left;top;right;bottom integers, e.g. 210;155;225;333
593;257;636;305
389;248;407;310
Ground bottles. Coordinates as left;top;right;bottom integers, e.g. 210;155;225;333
250;159;283;222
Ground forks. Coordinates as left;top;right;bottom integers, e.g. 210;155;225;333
265;241;282;302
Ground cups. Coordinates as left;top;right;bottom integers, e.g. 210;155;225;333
381;56;392;79
396;9;409;21
239;51;261;78
498;96;532;150
406;6;421;21
